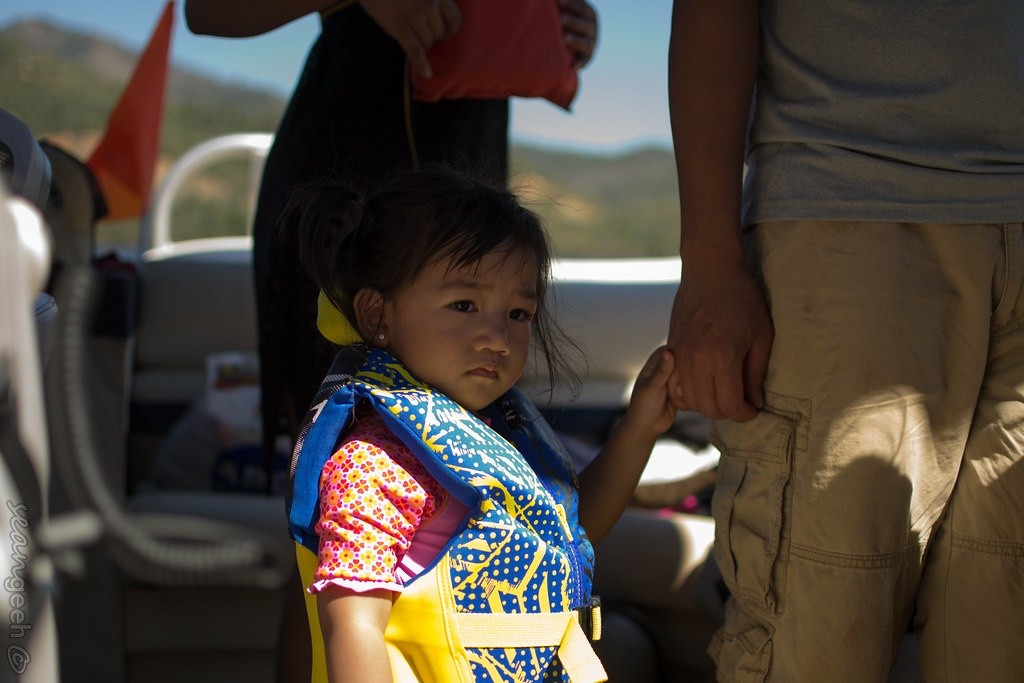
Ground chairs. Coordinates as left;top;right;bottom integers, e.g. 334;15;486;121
0;112;287;683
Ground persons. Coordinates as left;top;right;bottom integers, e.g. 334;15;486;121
662;0;1024;683
182;0;597;480
283;180;684;683
